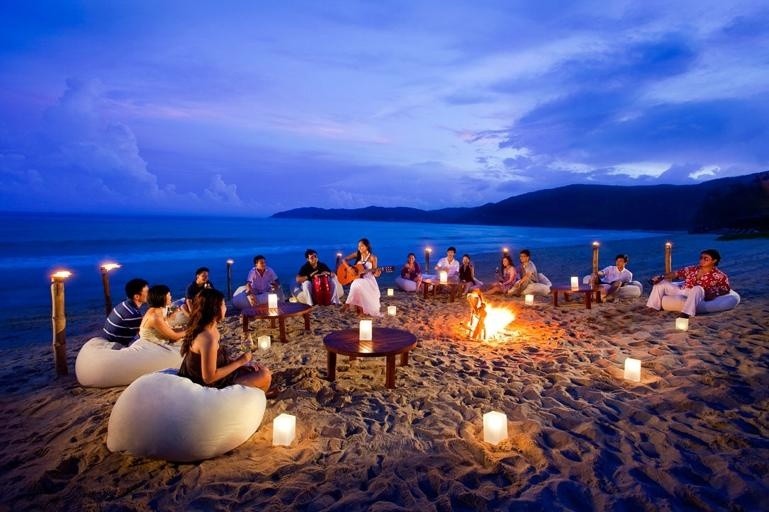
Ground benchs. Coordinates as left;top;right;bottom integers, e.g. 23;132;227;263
395;274;483;293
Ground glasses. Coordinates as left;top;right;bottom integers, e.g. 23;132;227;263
700;256;710;261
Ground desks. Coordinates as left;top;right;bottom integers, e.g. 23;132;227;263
553;284;604;309
323;326;417;389
240;301;313;341
421;279;467;302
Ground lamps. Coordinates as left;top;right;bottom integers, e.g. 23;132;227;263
571;276;579;288
388;288;397;316
359;318;373;341
268;294;278;310
624;356;642;383
483;411;509;445
676;317;689;331
526;294;534;305
256;335;271;348
272;412;296;446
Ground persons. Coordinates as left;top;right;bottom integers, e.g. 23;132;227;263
504;249;539;298
459;254;475;297
246;255;285;307
177;267;215;319
177;287;279;397
139;284;189;346
400;253;422;296
483;255;517;296
339;237;383;316
295;247;343;308
640;248;730;318
434;246;461;296
99;278;149;347
588;254;633;304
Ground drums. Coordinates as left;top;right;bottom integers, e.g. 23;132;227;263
314;273;332;305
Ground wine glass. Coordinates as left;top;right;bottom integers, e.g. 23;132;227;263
241;333;256;367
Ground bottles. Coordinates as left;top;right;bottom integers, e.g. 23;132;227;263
651;275;665;285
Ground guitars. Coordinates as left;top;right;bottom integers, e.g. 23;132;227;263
337;264;395;285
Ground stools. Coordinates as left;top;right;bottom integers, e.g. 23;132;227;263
660;281;742;315
521;272;553;295
74;335;267;465
583;274;643;302
